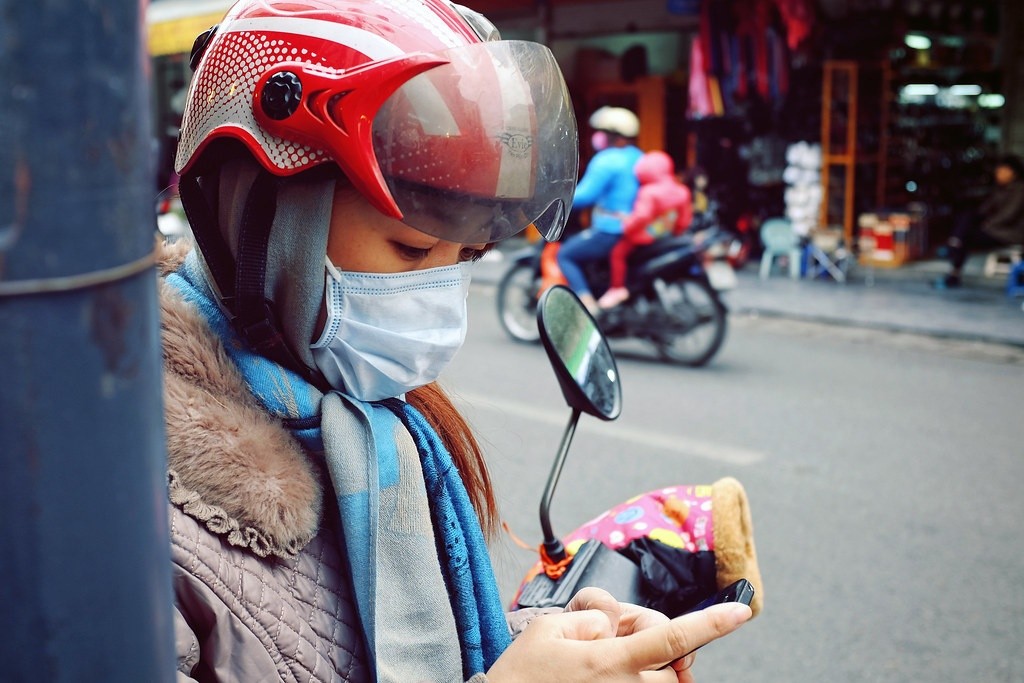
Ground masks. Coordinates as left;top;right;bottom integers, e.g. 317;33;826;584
308;255;473;402
591;131;608;151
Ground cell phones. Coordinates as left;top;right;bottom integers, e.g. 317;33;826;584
641;577;754;674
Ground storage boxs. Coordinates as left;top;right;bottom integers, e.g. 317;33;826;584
858;210;932;269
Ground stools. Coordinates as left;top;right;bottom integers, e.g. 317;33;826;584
985;243;1024;306
804;242;853;284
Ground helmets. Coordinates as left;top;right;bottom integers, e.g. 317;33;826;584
588;105;640;137
174;0;580;245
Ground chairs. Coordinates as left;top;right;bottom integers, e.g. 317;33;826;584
759;218;802;281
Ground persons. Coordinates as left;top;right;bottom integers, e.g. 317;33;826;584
558;105;693;320
928;153;1024;290
157;0;752;683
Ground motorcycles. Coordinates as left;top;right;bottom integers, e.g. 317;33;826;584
493;210;738;367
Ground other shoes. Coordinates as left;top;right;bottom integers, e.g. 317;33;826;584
578;293;599;316
598;287;629;308
